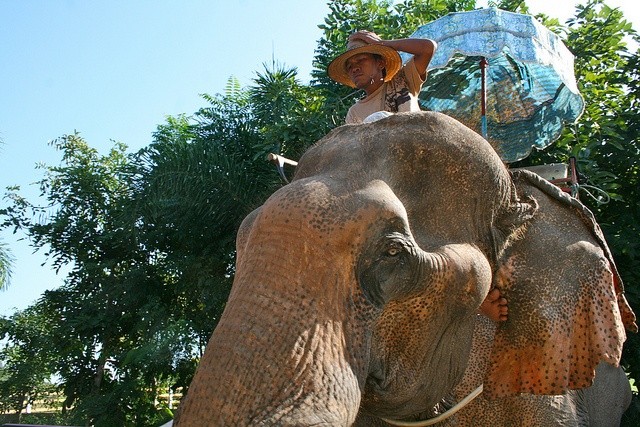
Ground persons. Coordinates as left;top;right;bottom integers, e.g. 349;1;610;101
326;29;439;124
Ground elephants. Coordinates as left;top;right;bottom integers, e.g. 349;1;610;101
171;110;640;426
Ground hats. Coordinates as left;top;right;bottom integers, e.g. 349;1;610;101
327;38;401;89
